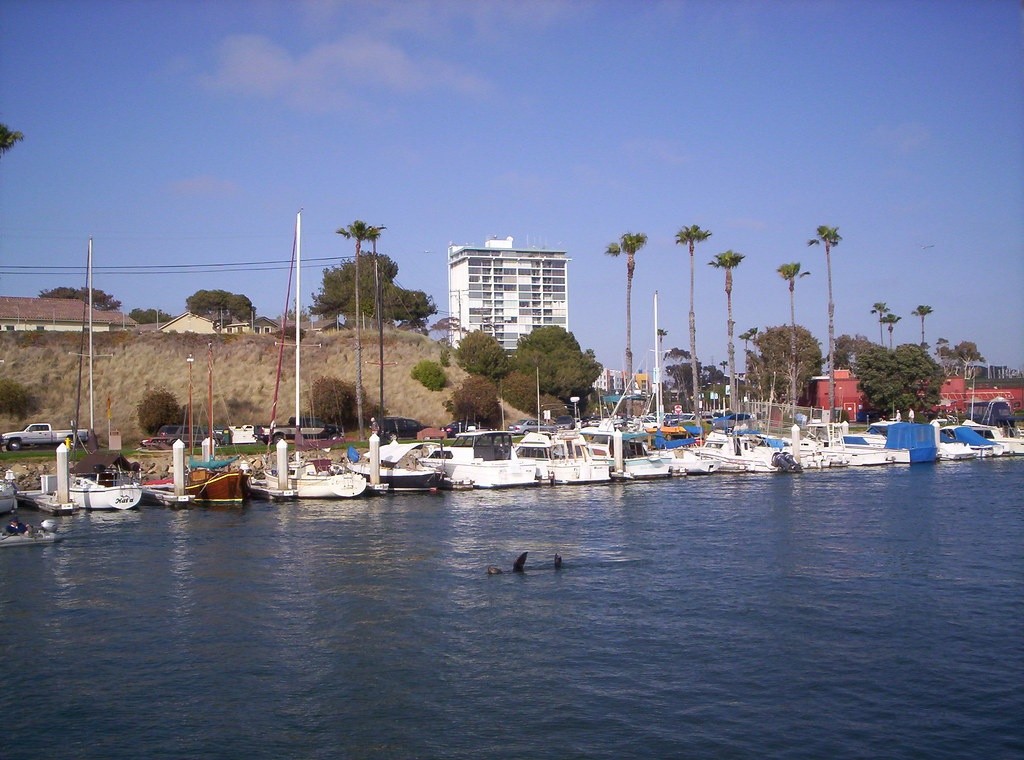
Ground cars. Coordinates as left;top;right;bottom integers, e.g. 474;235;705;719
555;414;576;430
714;413;750;428
508;418;559;437
440;418;498;439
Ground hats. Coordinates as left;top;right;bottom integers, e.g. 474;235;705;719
7;515;19;522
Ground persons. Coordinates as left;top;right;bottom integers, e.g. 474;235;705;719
369;417;380;435
909;407;915;423
6;516;31;536
896;410;902;422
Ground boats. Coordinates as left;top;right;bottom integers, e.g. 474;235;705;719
0;468;19;514
35;216;1024;515
0;519;65;548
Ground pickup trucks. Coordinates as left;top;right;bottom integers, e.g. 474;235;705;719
256;415;343;445
0;423;89;451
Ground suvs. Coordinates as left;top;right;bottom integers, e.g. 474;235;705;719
158;425;223;447
371;416;432;441
